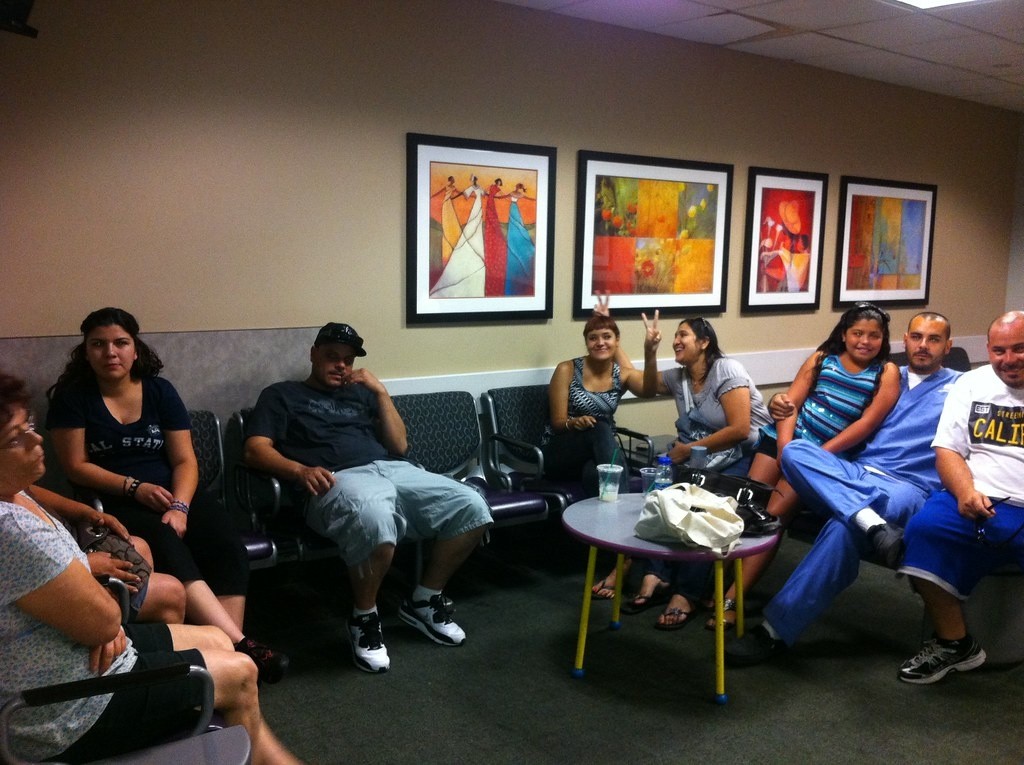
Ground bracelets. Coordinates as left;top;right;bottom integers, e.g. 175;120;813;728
170;499;190;514
126;479;140;499
565;419;570;431
123;476;130;495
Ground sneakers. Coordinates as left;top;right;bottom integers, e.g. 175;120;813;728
346;607;391;673
898;637;986;684
236;636;290;683
398;594;466;646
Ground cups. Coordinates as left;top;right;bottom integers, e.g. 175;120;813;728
597;464;623;502
639;467;663;497
689;445;707;469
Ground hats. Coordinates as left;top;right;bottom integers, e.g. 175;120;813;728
314;322;366;357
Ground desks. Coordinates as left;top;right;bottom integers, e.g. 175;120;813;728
617;435;676;468
563;493;780;705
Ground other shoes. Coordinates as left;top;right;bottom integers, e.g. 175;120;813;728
867;522;906;563
724;624;786;665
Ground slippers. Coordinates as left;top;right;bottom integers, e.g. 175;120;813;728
621;591;655;614
705;600;745;630
654;605;698;629
591;577;616;599
702;589;716;608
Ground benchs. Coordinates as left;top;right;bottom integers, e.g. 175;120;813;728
787;510;1024;669
49;386;651;592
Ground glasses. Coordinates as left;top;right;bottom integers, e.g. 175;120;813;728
0;413;36;451
696;316;709;336
852;302;891;324
974;497;1024;551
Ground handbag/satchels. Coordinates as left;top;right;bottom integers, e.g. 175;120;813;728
633;482;744;559
65;517;151;625
672;465;782;538
675;367;743;473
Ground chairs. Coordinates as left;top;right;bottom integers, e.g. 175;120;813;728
0;574;251;765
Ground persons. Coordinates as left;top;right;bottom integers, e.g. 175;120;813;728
45;307;291;685
22;483;186;625
706;302;964;666
0;375;301;765
593;290;775;630
543;309;661;599
896;310;1024;685
242;323;495;674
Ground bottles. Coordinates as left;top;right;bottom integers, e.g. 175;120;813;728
654;457;673;490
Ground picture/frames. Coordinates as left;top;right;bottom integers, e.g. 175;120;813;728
740;166;829;313
832;176;938;309
404;131;557;324
573;149;734;318
